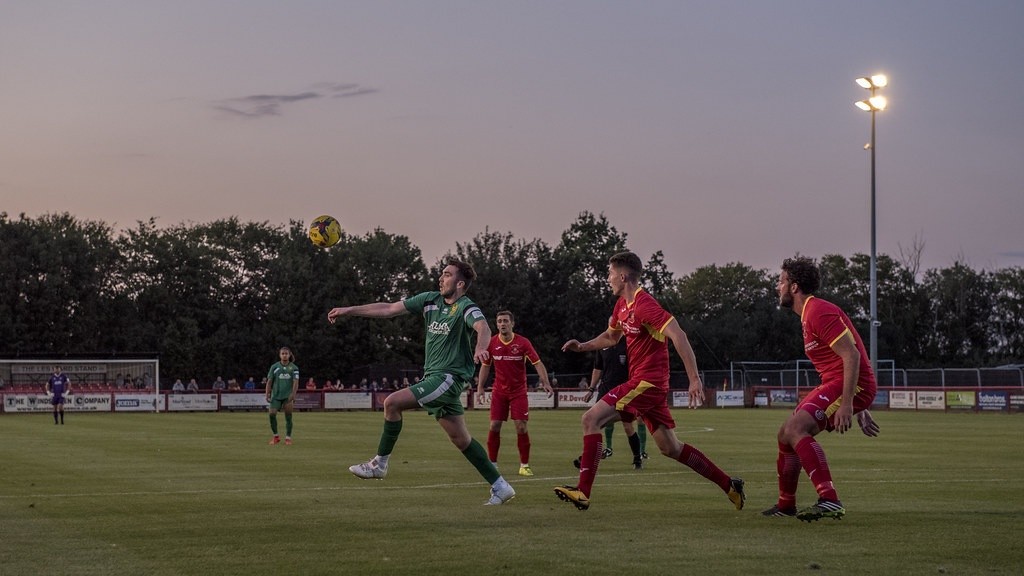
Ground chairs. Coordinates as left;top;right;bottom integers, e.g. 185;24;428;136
0;384;154;395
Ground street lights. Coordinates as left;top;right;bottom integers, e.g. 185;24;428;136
854;74;893;409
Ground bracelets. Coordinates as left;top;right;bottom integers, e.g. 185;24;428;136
588;387;595;392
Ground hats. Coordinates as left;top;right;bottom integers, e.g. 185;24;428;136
217;377;221;381
383;378;387;382
249;377;253;382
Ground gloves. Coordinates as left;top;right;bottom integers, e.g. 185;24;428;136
47;391;54;398
64;390;70;399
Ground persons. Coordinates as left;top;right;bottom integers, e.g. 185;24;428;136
477;311;553;476
554;253;747;511
173;378;198;413
328;263;515;505
266;347;299;445
573;315;649;471
45;366;71;425
116;372;152;389
213;376;267;412
579;377;588;392
323;379;344;412
761;260;880;523
350;375;557;412
306;378;316;412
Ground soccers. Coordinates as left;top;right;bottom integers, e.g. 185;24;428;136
309;216;342;247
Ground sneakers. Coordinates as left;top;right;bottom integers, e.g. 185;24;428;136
483;483;515;505
600;448;613;459
632;457;643;469
519;465;533;476
349;458;387;480
795;498;846;523
285;439;292;445
574;456;582;467
726;478;747;511
554;485;590;511
269;435;280;445
760;503;799;517
640;453;650;461
